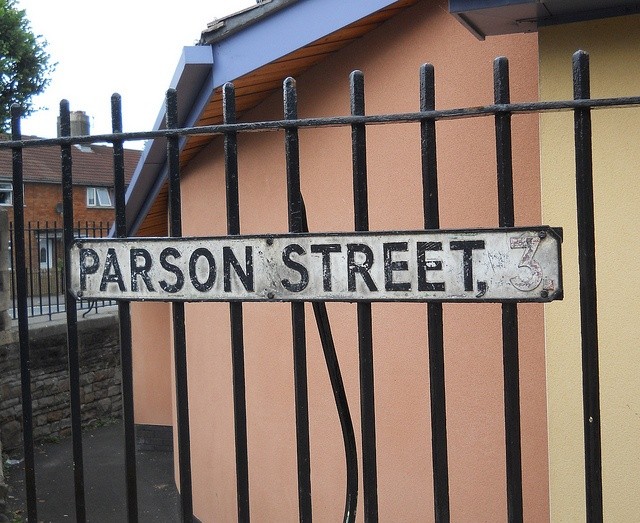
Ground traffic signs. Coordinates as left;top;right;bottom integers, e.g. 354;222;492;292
66;226;563;304
